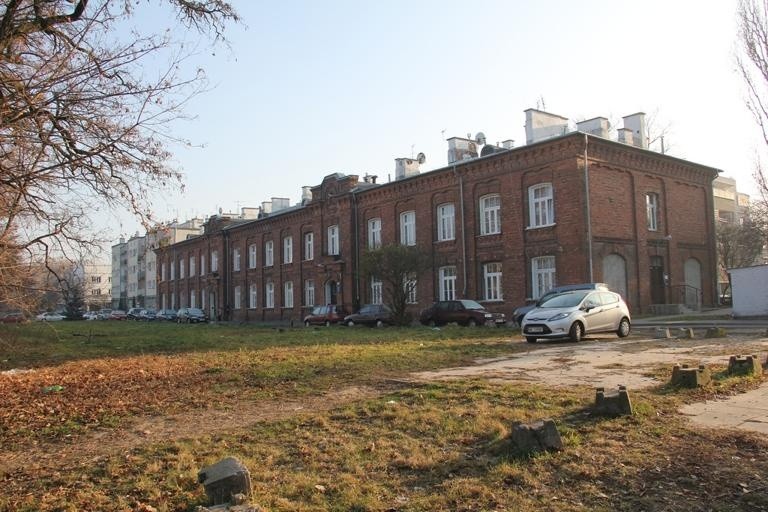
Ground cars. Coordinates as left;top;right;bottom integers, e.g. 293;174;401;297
0;308;206;324
